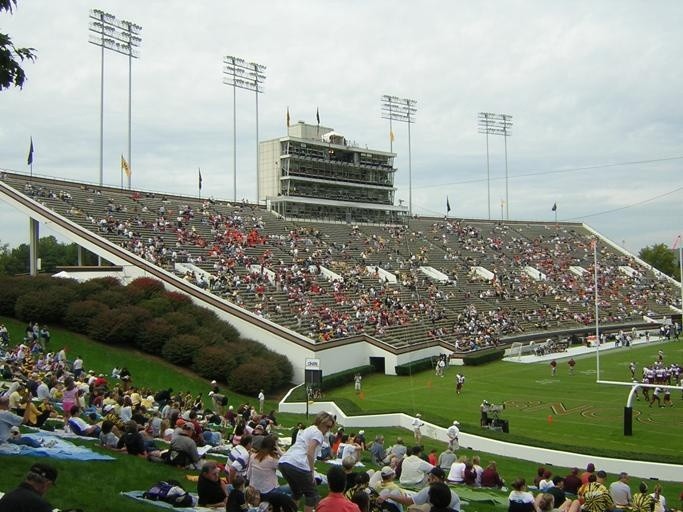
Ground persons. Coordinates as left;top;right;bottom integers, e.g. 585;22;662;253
412;413;424;442
354;373;361;390
508;463;668;512
629;360;683;408
480;399;498;424
568;358;578;375
1;463;65;512
435;357;446;377
258;390;265;413
276;412;334;512
227;403;304;501
318;427;463;512
18;181;681;357
225;476;297;512
447;421;461;452
550;359;558;377
0;317;241;471
439;446;506;488
456;372;464;395
195;462;230;506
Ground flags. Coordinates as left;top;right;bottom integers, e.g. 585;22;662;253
389;126;395;142
316;108;319;123
286;109;290;127
199;171;202;189
123;159;131;176
27;139;34;166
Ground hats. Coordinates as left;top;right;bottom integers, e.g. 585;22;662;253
104;404;114;412
30;464;58;489
176;419;196;433
426;469;445;479
381;466;395;476
553;476;565;482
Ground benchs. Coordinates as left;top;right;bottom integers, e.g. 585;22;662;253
1;170;186;284
186;202;682;354
